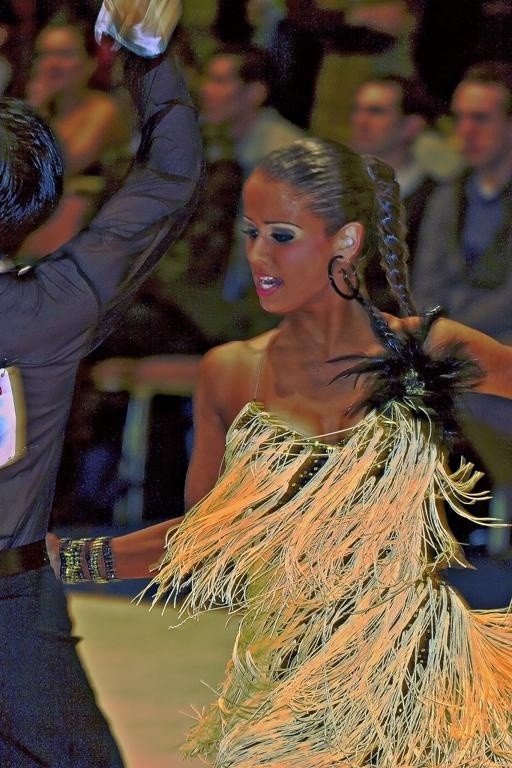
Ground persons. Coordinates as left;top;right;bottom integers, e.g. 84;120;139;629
1;0;511;612
0;1;201;768
46;138;510;764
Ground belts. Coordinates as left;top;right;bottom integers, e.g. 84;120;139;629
1;540;50;577
